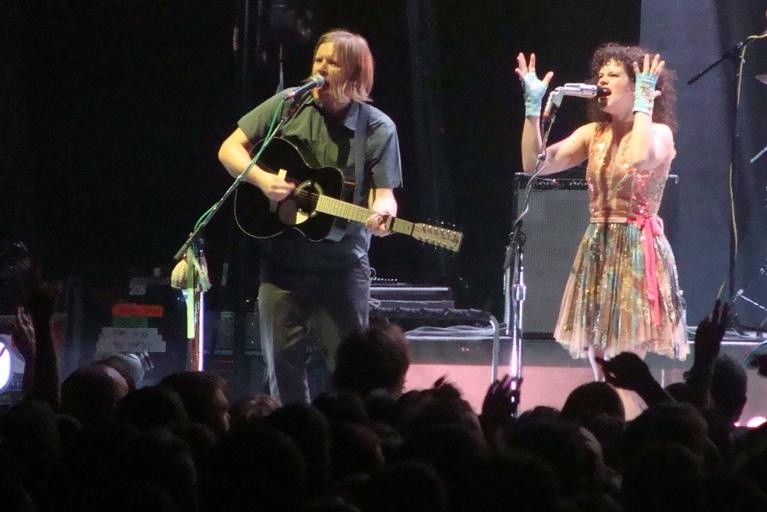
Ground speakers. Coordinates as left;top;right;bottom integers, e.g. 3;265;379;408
513;178;589;339
726;268;767;330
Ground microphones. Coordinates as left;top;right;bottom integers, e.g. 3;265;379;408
283;75;325;100
555;82;603;99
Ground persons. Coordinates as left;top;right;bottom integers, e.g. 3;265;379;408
212;31;404;417
0;251;766;511
514;38;688;419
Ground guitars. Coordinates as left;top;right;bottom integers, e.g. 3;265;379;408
234;135;463;254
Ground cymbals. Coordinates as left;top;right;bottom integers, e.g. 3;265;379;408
754;74;766;86
